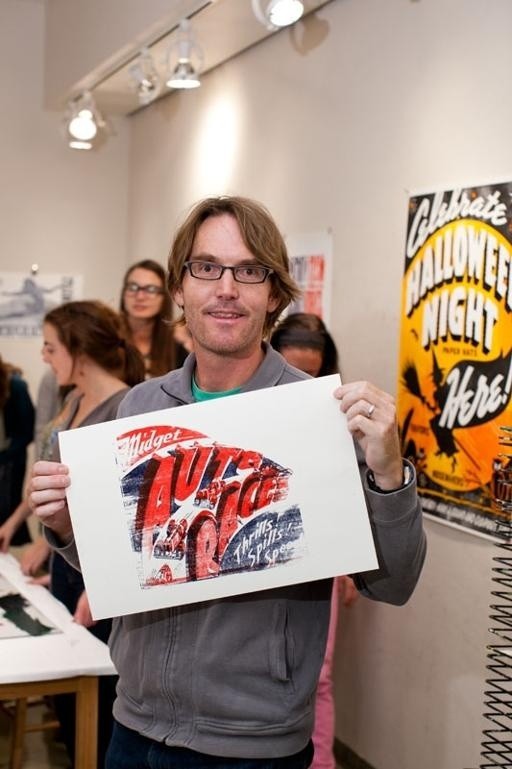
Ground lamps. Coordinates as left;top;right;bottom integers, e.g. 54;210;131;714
68;1;304;151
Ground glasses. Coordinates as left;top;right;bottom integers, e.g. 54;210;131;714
184;259;275;284
125;283;164;298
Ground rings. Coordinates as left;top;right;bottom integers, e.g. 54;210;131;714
365;402;376;419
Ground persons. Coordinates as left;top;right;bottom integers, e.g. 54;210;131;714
268;313;360;767
26;190;430;767
116;255;188;381
24;297;147;766
0;343;75;574
0;355;38;552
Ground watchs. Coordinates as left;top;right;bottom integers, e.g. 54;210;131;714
368;465;413;492
